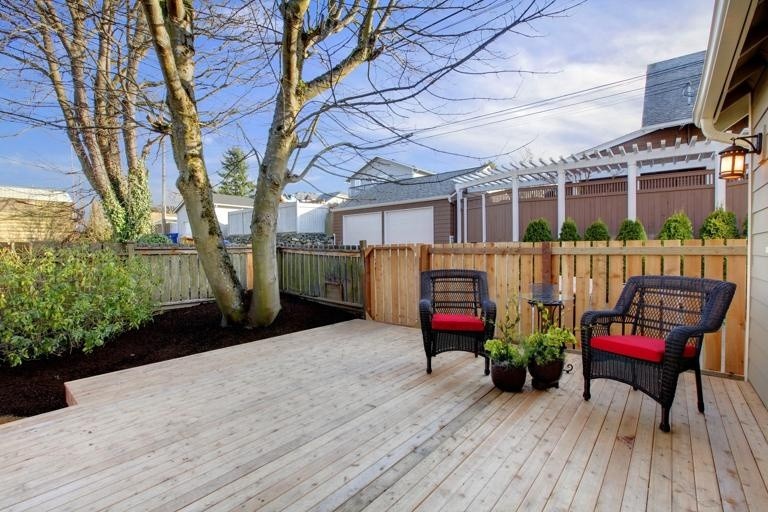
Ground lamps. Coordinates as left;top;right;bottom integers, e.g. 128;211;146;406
716;133;762;180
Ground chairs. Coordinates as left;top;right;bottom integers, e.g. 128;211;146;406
580;274;737;432
418;268;496;377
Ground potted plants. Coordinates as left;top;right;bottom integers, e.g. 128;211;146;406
483;284;578;393
